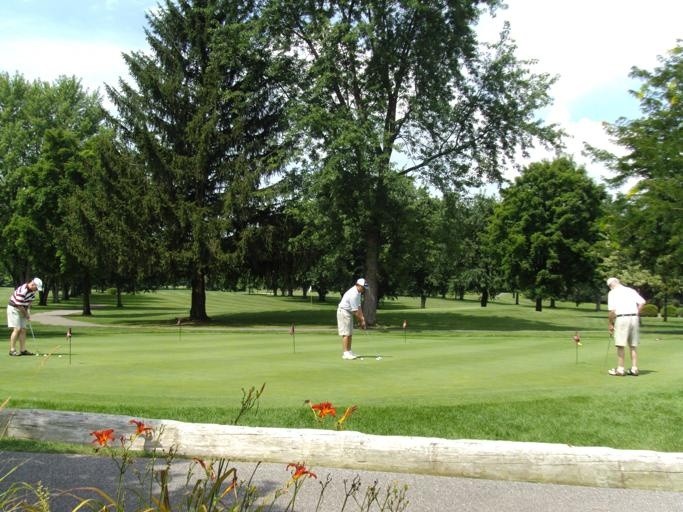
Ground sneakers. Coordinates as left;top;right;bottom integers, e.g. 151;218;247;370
7;350;21;356
20;350;36;356
341;353;359;360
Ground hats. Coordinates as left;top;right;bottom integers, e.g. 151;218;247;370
355;278;369;291
606;277;617;288
32;277;43;292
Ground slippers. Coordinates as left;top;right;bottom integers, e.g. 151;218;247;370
625;369;639;376
606;368;625;376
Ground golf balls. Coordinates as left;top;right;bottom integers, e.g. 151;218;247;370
376;357;380;360
361;357;365;360
43;354;65;358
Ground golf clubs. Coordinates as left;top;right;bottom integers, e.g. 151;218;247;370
29;320;40;356
364;326;382;361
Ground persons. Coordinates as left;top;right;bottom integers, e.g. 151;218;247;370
337;279;370;359
607;277;647;376
6;277;43;356
